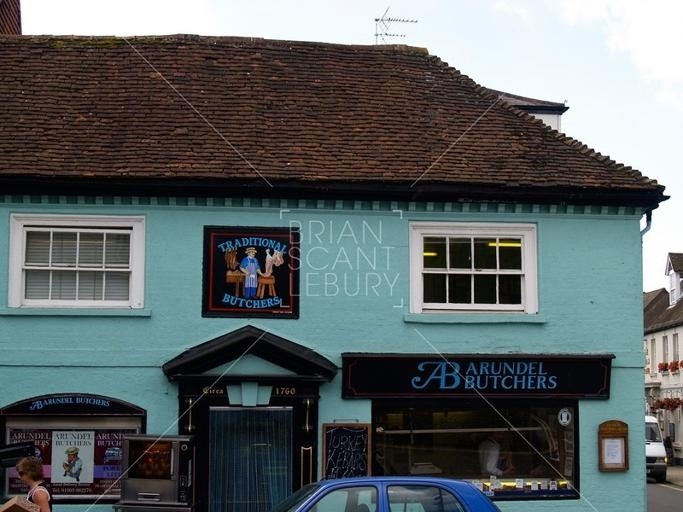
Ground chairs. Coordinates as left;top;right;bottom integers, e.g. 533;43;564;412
350;495;460;512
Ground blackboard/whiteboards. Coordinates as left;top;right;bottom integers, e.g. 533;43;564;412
663;437;674;467
322;423;371;480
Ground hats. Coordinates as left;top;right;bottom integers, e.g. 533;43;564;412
245;248;257;253
65;447;79;454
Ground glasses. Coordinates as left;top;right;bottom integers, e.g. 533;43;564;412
18;470;25;477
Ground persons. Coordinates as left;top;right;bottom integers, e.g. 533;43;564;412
238;247;264;300
474;423;516;477
14;455;51;511
61;446;82;481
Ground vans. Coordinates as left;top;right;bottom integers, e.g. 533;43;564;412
645;415;668;485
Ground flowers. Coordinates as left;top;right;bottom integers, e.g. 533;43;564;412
658;361;680;372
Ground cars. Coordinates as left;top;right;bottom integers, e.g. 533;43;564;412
271;475;505;512
